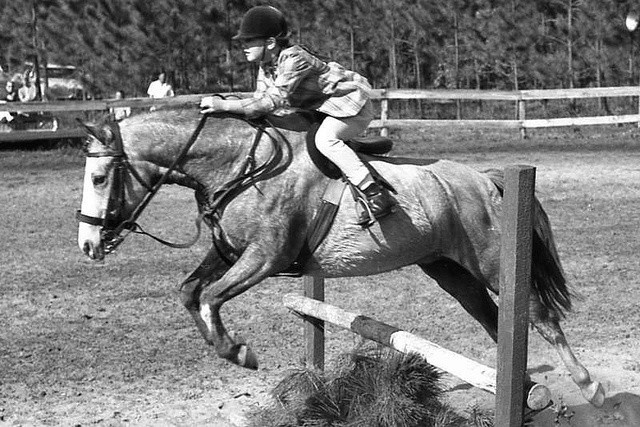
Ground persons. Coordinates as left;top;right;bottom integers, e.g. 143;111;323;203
109;90;131;121
18;75;37;102
200;6;395;225
2;80;18;102
147;72;174;111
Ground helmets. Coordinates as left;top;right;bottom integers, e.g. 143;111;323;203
232;6;288;41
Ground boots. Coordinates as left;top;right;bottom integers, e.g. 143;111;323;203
355;186;399;222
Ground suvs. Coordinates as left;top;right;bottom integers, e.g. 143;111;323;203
21;62;89;100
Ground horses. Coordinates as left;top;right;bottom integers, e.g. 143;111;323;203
75;99;606;409
